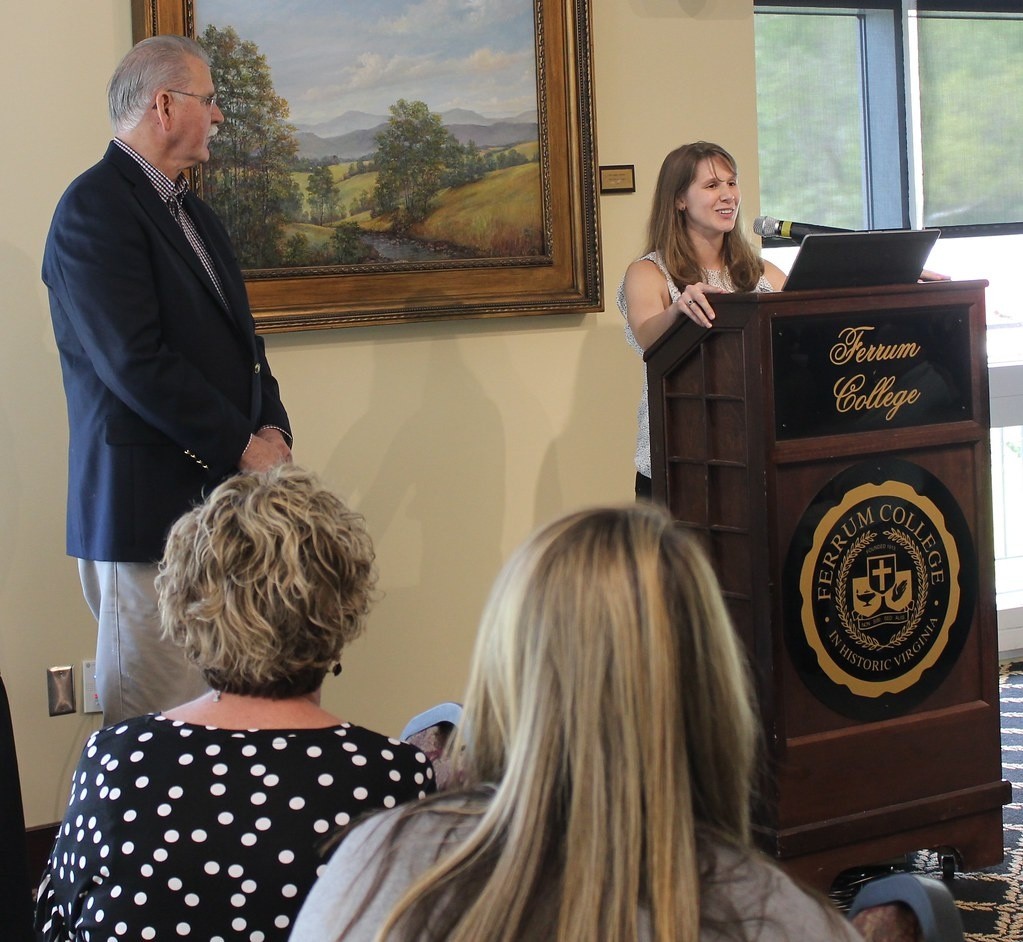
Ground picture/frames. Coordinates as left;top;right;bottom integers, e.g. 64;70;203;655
126;0;605;335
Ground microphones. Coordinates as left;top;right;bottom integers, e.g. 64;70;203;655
754;215;854;246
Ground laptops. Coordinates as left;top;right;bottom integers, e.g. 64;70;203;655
780;229;941;293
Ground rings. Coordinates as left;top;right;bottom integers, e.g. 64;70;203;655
687;300;693;307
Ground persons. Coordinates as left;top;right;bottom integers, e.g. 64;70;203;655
41;37;293;727
290;504;860;942
618;142;788;505
31;467;436;942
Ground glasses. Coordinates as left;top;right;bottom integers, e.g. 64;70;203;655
152;90;219;109
